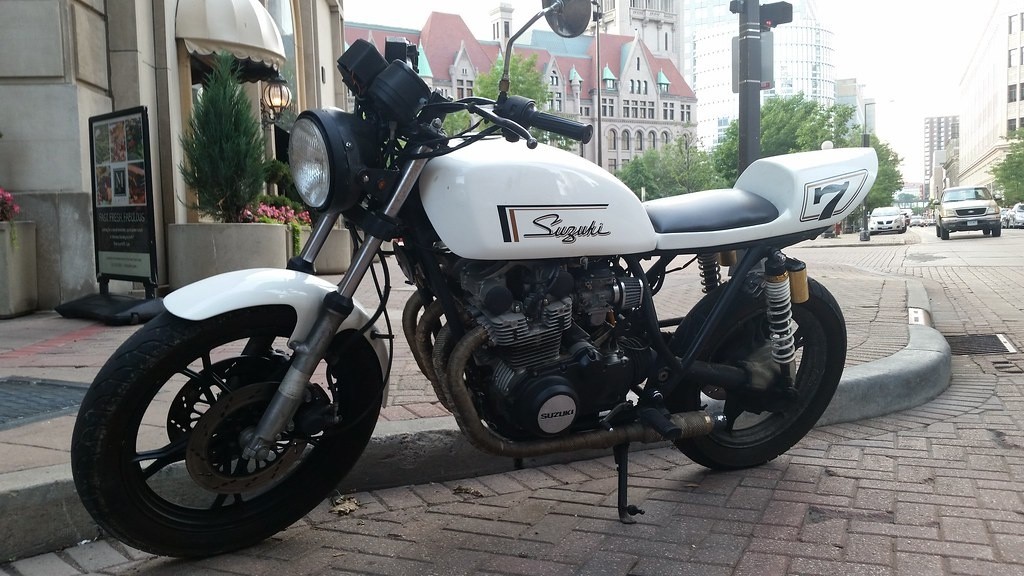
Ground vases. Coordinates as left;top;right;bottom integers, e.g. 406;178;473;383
285;224;380;275
0;220;38;319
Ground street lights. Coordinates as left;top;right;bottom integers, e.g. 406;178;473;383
864;101;876;241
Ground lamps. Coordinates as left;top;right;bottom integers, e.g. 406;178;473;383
260;69;292;130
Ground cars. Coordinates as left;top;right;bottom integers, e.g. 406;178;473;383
1000;202;1024;229
867;207;913;235
933;185;1002;241
909;216;924;228
924;218;936;226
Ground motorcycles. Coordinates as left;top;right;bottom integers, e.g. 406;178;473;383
70;0;879;558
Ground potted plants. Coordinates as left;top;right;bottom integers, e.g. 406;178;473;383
168;49;290;292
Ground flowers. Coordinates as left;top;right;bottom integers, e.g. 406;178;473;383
237;199;312;224
0;187;23;222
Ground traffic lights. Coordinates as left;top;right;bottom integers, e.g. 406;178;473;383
758;1;794;28
